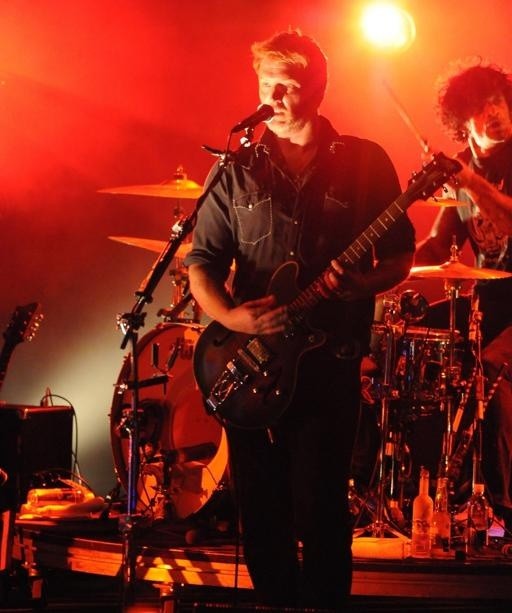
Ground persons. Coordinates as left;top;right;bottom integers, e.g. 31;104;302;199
181;30;415;613
407;61;512;551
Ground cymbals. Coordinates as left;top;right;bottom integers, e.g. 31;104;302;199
414;196;466;206
107;234;195;259
408;260;511;281
96;180;204;201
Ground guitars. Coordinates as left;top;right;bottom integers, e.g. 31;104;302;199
190;152;461;426
1;301;43;391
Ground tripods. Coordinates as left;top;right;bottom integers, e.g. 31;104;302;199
352;281;512;555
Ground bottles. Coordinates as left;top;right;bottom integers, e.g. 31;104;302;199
412;469;492;564
28;488;95;509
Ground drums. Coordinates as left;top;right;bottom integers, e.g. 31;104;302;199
363;322;460;422
111;318;230;524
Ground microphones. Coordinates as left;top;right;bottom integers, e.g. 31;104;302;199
226;103;274;135
